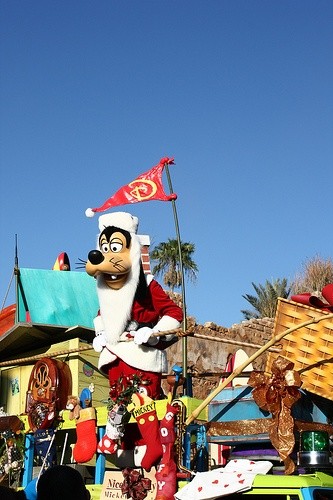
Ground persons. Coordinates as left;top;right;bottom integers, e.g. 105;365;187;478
1;439;22;469
37;465;91;500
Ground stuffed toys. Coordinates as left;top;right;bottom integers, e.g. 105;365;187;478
66;396;82;420
75;211;184;467
